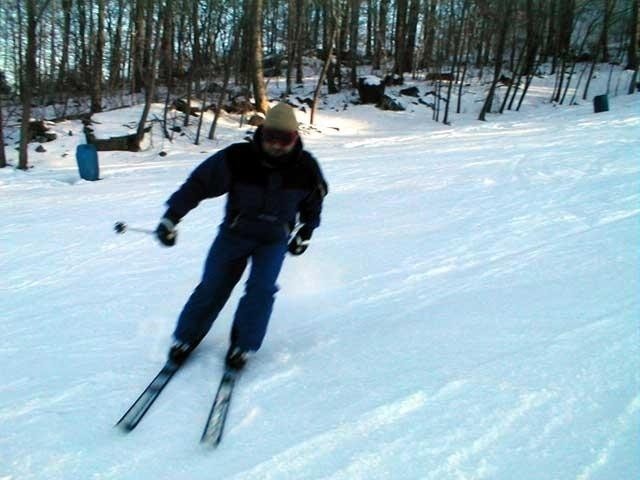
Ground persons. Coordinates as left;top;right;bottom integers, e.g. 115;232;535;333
151;102;329;374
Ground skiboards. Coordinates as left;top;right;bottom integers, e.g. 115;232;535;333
113;359;238;451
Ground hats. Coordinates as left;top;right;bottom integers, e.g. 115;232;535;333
262;102;298;131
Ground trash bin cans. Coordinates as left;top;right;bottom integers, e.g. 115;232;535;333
594;95;610;111
77;145;98;181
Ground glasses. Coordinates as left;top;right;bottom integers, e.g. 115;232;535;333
261;128;297;146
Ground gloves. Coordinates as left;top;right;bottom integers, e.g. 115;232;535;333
157;209;179;246
287;225;314;255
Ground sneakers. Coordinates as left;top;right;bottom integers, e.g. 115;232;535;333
226;345;250;370
168;338;192;364
